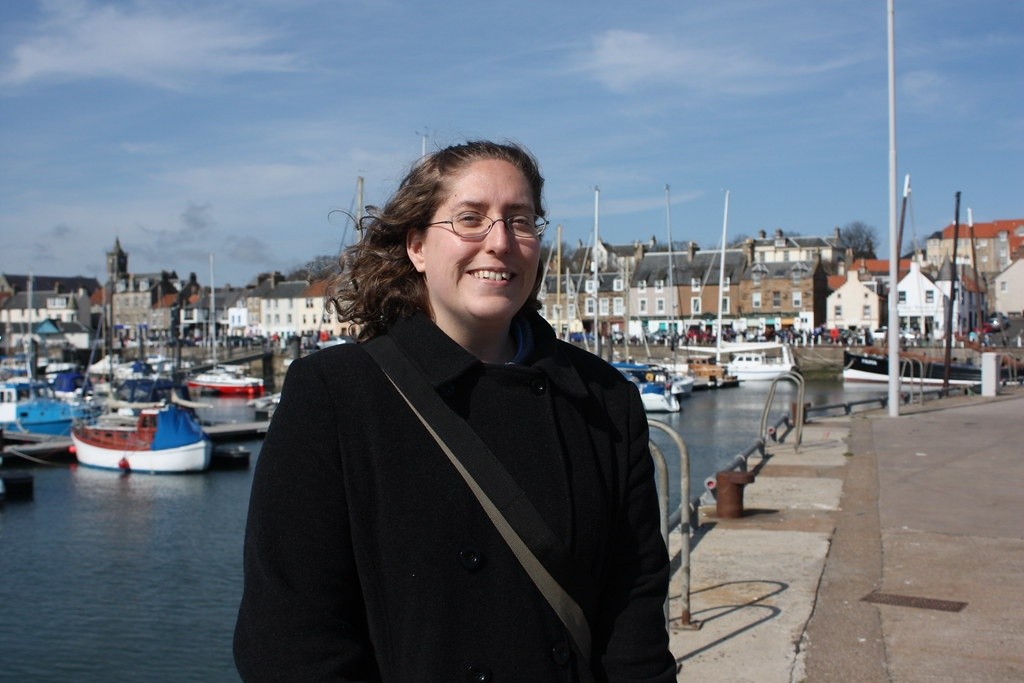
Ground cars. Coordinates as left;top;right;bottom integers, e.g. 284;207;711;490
971;322;995;335
988;309;1011;331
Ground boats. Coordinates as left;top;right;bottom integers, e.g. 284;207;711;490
0;180;801;472
839;349;1024;384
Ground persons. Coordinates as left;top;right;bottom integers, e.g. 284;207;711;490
614;317;875;348
233;141;677;683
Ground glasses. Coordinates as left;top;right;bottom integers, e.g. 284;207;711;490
427;211;549;239
